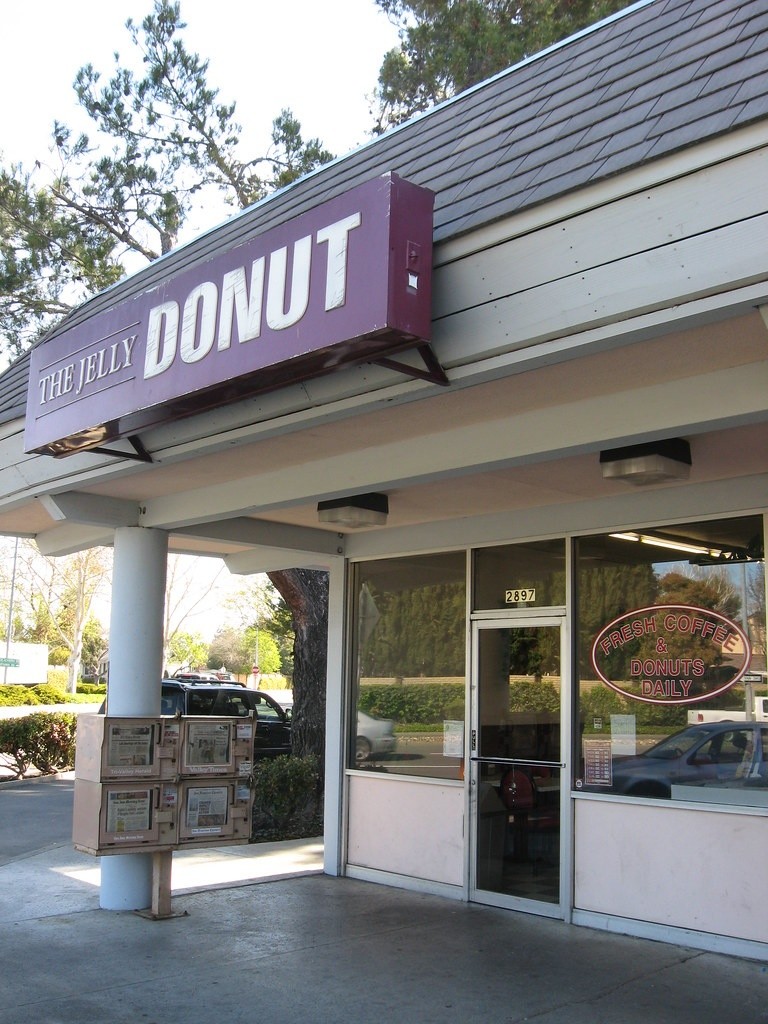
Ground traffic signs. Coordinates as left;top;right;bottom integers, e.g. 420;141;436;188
735;673;764;683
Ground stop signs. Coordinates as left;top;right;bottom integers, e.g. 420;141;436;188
252;666;259;674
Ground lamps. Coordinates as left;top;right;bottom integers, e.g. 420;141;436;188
600;439;692;487
316;493;389;528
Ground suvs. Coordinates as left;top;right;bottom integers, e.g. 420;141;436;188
95;678;294;761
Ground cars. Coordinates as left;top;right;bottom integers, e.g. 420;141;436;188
576;716;768;801
175;667;294;719
354;709;401;763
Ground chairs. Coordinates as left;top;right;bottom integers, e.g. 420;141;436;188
161;700;169;714
500;768;560;869
228;701;241;717
731;737;747;763
